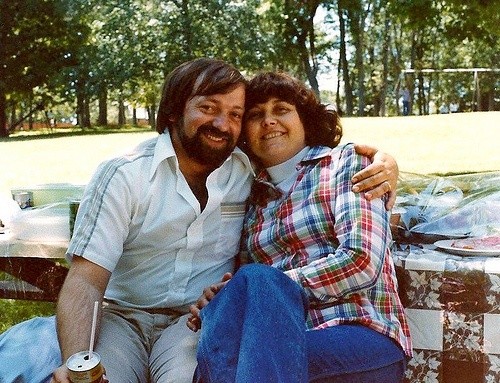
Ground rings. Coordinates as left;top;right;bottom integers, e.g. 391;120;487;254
385;181;392;193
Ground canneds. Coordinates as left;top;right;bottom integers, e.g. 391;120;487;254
66;350;103;383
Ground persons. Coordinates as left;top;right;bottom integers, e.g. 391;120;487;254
47;59;399;383
402;83;412;115
449;100;459;113
438;102;448;114
187;71;413;383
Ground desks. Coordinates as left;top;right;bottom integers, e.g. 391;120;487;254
0;233;72;302
